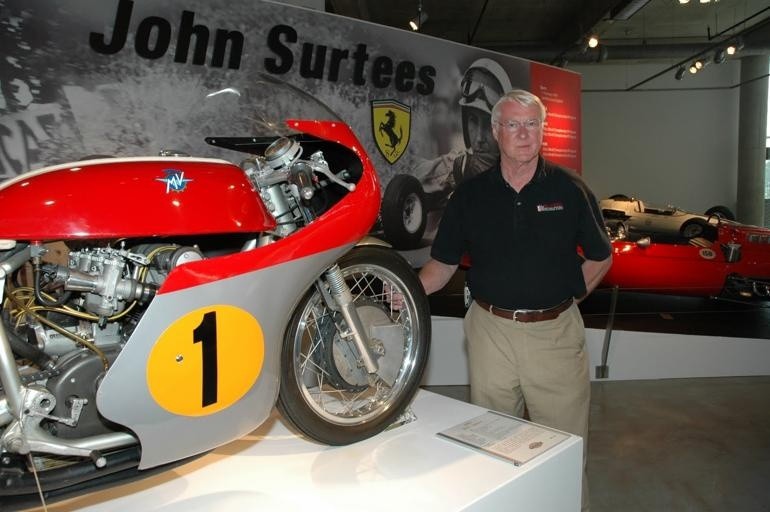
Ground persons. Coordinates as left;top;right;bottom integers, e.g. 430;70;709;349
384;88;615;512
413;57;512;240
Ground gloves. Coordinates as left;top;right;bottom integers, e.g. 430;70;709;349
453;153;498;184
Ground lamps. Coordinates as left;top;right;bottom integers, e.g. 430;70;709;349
408;11;430;31
578;34;600;49
675;40;744;80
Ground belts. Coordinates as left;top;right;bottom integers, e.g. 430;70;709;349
474;296;573;322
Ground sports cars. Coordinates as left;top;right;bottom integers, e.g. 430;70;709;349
370;144;465;252
597;193;756;245
573;210;770;302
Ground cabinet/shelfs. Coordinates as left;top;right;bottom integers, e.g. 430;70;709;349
29;386;584;512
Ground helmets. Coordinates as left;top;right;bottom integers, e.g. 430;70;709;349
458;58;513;148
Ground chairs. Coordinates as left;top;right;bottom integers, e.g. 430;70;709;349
608;219;653;246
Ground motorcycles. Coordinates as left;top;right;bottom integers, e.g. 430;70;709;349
0;63;438;511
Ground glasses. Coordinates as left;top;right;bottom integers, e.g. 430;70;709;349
461;67;505;111
497;120;541;129
